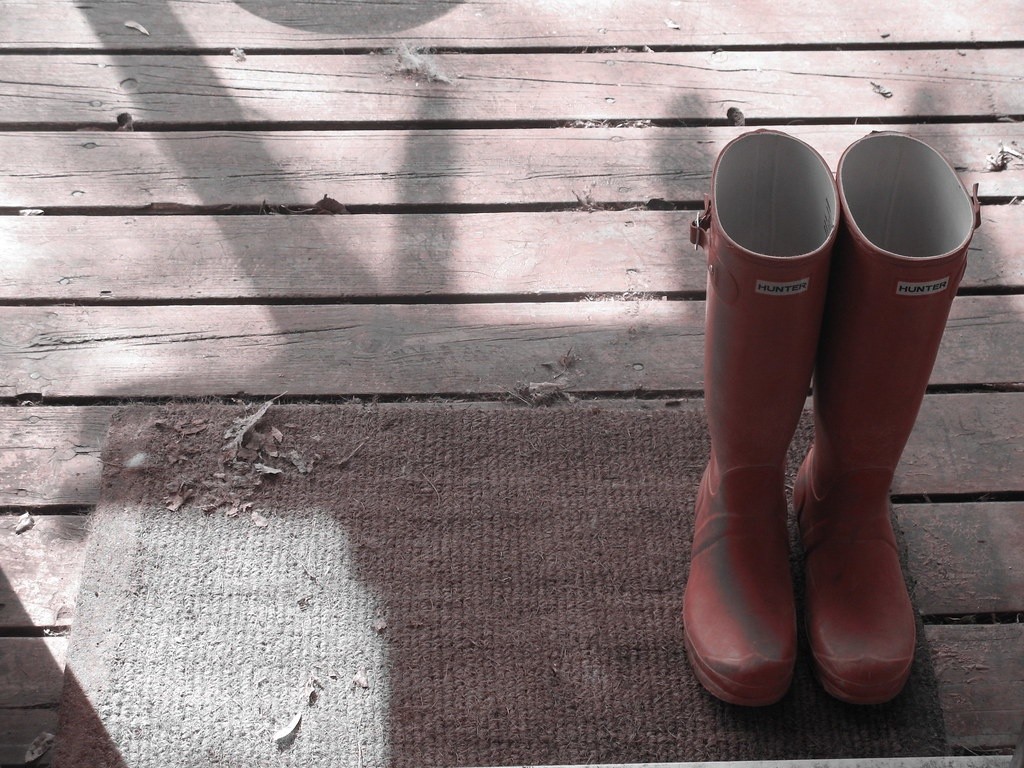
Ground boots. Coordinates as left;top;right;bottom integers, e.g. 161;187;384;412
683;130;841;707
794;133;981;706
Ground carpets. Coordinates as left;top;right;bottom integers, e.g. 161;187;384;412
48;405;948;768
